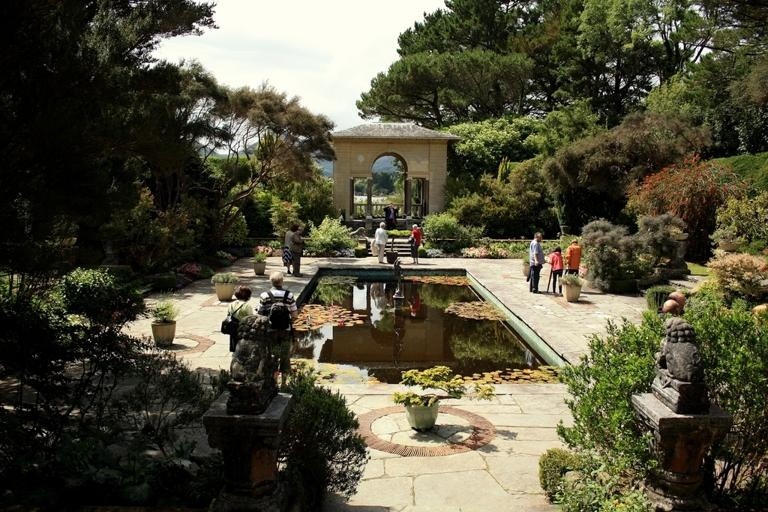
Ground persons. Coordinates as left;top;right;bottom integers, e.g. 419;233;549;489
255;270;297;375
409;224;421;264
548;246;563;297
392;342;403;369
563;238;584;276
290;227;305;277
374;221;387;263
407;291;421;317
371;283;387;315
382;202;398;230
528;231;545;293
227;286;254;353
281;224;303;274
393;257;403;296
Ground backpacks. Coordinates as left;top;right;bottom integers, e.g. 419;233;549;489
265;289;290;330
220;302;248;334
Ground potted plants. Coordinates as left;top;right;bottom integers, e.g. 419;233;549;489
392;362;502;430
253;253;268;276
559;273;582;302
211;270;242;301
149;303;179;346
520;255;531;278
385;229;401;263
